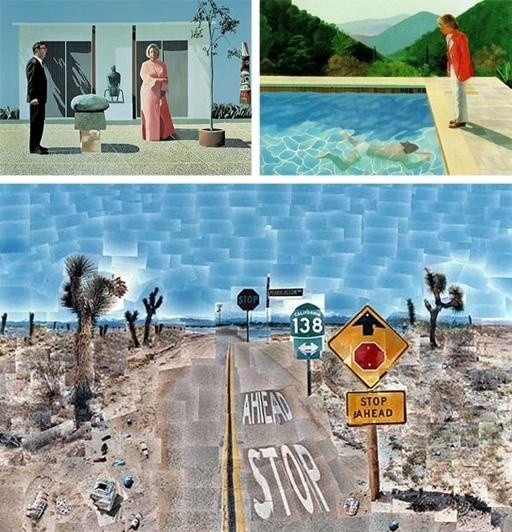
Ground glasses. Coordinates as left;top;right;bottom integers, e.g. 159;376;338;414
40;47;48;52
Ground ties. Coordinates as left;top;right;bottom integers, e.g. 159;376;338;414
41;62;45;69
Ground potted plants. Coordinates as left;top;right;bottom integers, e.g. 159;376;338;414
190;0;241;147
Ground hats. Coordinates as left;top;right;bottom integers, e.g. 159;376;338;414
145;43;160;58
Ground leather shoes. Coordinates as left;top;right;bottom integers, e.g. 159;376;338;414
30;144;50;155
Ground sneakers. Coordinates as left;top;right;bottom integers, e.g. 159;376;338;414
448;119;466;129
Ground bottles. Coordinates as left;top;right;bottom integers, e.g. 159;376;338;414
130;517;140;529
124;476;133;489
390;521;399;530
29;493;48;522
140;442;149;460
